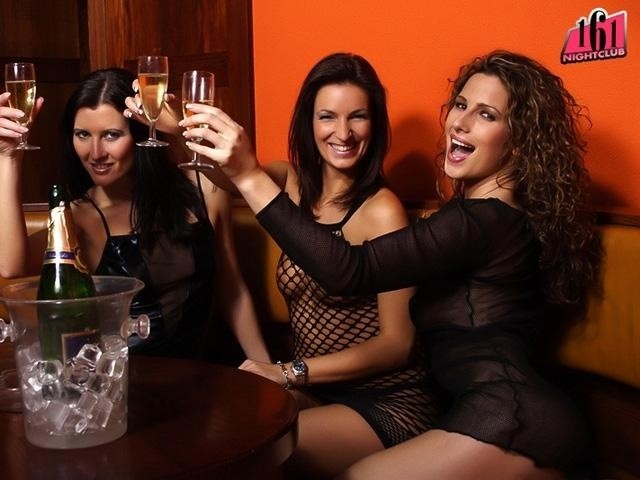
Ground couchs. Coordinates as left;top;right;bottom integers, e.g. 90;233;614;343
1;195;640;480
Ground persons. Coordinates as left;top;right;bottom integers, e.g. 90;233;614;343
122;52;434;480
178;50;606;480
0;68;273;364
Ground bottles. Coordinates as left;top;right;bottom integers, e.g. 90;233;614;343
37;184;99;364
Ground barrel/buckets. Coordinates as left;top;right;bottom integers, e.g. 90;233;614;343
0;277;149;450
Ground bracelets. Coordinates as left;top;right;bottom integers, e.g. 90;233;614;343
277;360;293;390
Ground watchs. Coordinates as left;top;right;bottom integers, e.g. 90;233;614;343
291;359;308;386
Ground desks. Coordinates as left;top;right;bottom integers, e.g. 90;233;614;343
1;355;303;480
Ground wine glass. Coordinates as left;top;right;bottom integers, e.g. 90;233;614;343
139;54;214;171
4;63;41;153
